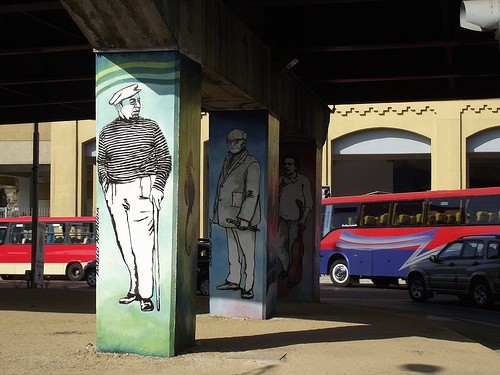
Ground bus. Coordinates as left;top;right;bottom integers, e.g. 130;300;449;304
0;216;96;282
319;186;500;289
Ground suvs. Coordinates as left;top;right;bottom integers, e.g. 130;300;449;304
405;232;500;308
83;237;210;297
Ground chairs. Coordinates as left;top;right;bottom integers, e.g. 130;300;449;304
22;238;29;244
348;208;500;226
